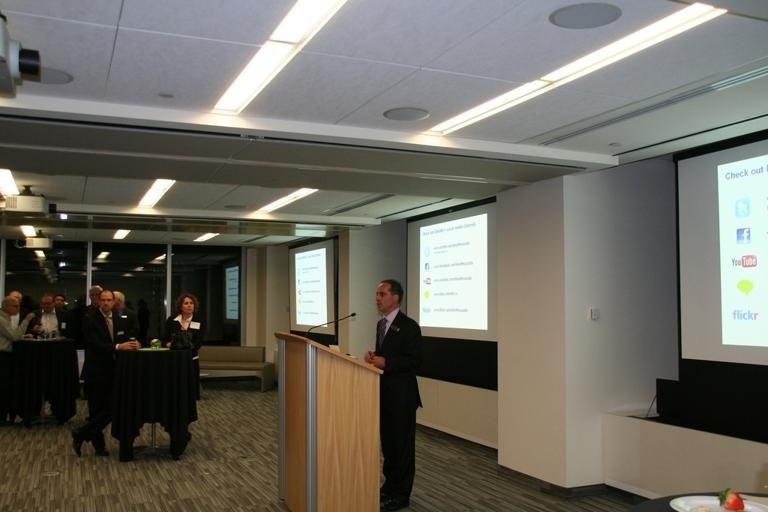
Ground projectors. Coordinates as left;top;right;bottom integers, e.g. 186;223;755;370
0;14;40;98
14;236;53;248
0;185;58;214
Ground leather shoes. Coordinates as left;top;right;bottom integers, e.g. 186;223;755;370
72;431;83;456
380;487;409;512
95;449;108;456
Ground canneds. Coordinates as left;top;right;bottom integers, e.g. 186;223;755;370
128;337;136;341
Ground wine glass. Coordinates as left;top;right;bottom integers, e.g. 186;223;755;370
43;328;48;340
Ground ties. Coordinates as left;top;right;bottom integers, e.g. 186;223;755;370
378;318;387;345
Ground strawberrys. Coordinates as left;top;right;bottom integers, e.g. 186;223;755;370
718;487;744;512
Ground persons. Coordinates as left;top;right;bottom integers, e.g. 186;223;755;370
163;293;206;462
1;285;150;456
362;279;423;511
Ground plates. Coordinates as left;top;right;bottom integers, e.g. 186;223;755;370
670;496;768;512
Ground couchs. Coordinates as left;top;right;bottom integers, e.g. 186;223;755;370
198;345;276;392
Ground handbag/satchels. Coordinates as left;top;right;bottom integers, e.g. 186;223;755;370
170;332;192;349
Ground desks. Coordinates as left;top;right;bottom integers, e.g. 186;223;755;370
113;346;196;457
11;335;74;425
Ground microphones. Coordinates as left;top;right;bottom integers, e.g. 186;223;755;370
305;313;356;343
